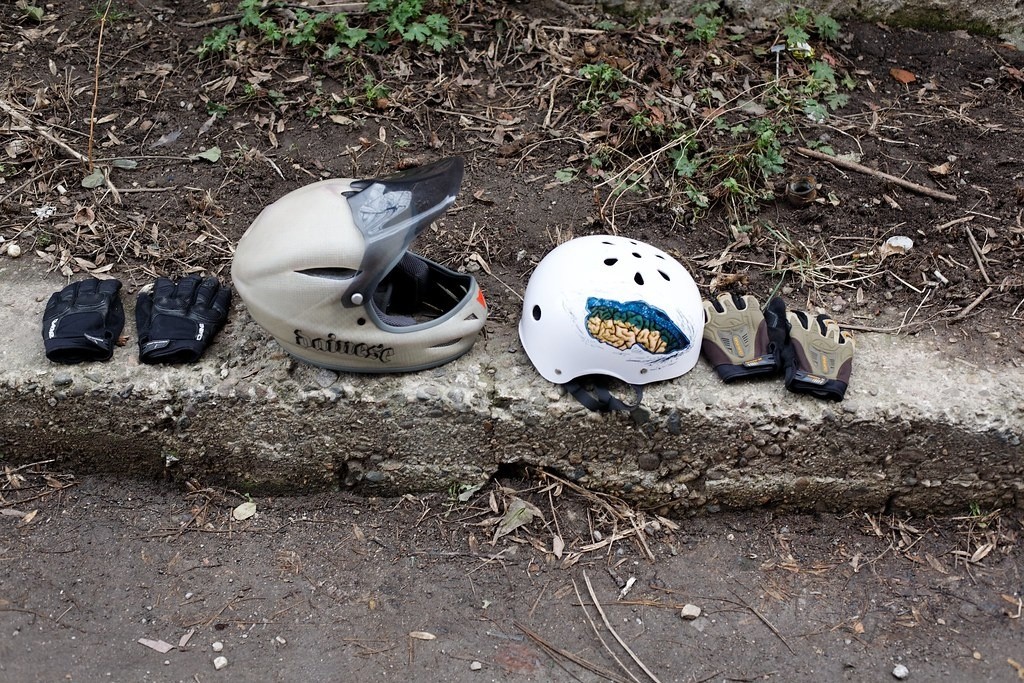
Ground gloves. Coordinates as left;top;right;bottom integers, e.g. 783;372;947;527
41;278;126;363
702;292;775;382
135;274;230;363
768;296;855;402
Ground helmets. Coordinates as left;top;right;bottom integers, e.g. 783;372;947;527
518;234;705;384
231;154;488;374
